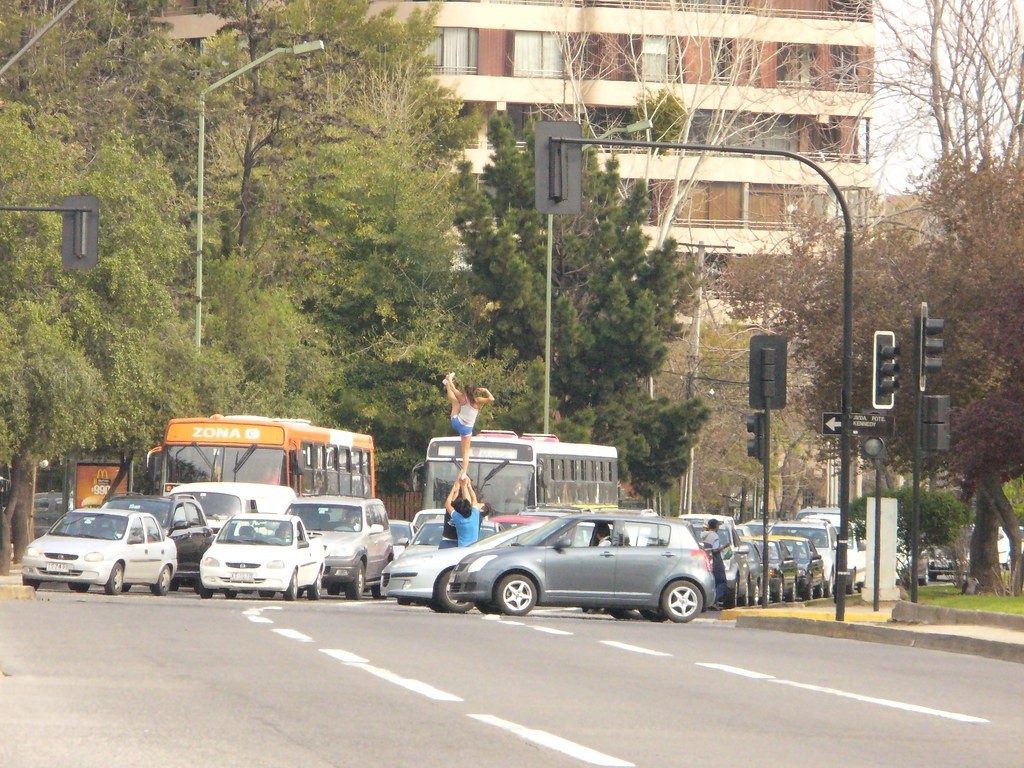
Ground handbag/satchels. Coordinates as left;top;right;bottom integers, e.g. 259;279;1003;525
722;546;732;560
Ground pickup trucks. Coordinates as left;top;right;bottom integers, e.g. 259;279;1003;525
200;511;326;601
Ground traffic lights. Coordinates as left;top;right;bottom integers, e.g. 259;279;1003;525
919;302;944;392
871;330;900;409
747;412;765;464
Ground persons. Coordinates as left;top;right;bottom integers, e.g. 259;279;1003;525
594;522;612;547
263;522;292;544
700;519;730;611
253;460;279;484
505;477;529;504
442;372;495;481
438;469;491;548
340;511;361;532
110;519;142;544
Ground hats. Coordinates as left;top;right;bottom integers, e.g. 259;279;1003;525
708;519;723;525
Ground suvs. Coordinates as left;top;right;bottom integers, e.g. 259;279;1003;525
99;491;216;594
767;519;839;598
679;513;752;608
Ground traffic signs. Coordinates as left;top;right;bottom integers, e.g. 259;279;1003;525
821;412;896;437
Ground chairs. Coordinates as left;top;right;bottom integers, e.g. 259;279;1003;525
239;526;255;537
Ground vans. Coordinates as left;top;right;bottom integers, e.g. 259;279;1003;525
794;507;841;520
803;515;867;594
170;481;295;526
284;493;393;600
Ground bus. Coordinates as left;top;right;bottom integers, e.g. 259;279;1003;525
411;430;619;517
142;413;375;502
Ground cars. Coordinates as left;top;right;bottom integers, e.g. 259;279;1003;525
444;512;715;624
895;525;1024;587
738;536;824;601
380;507;548;605
20;507;178;596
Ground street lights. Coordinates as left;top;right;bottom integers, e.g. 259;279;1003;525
543;118;653;436
195;38;325;354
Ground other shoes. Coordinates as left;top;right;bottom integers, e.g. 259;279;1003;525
711;604;720;611
442;373;455;385
